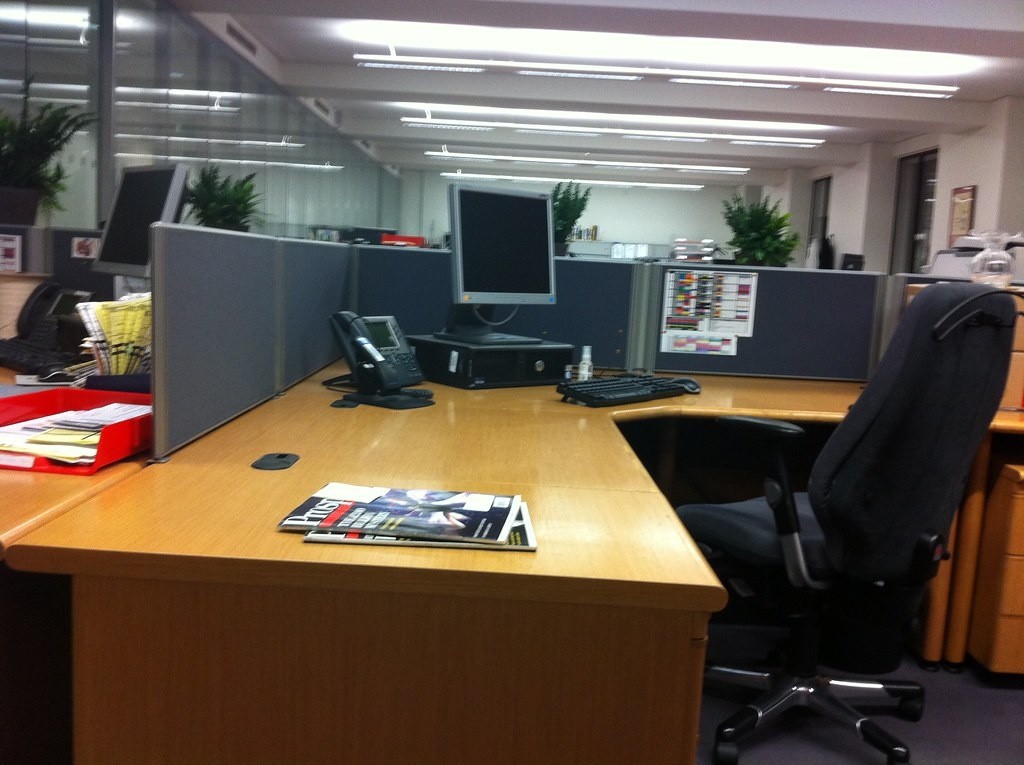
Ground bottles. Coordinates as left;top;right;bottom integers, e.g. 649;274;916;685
579;346;593;382
970;230;1012;290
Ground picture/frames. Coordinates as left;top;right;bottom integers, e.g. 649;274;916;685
947;185;975;251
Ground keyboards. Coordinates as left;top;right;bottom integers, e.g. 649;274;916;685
0;338;78;375
556;375;686;407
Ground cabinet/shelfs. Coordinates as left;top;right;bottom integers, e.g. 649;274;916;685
968;460;1024;675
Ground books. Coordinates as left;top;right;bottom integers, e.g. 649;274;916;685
275;483;536;553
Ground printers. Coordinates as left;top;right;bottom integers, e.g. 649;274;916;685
921;236;1024;283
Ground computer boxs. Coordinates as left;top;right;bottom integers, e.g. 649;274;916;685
402;334;574;389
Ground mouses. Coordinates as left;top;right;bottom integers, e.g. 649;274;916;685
670;378;701;394
38;363;74;382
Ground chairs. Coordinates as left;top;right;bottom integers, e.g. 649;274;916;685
671;280;1017;765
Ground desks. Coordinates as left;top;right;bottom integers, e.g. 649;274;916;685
3;368;1024;765
0;362;153;565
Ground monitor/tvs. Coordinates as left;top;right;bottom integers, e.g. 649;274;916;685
353;226;398;245
433;183;557;346
93;163;191;295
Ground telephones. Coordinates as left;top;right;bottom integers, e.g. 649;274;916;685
16;277;96;354
328;310;425;392
